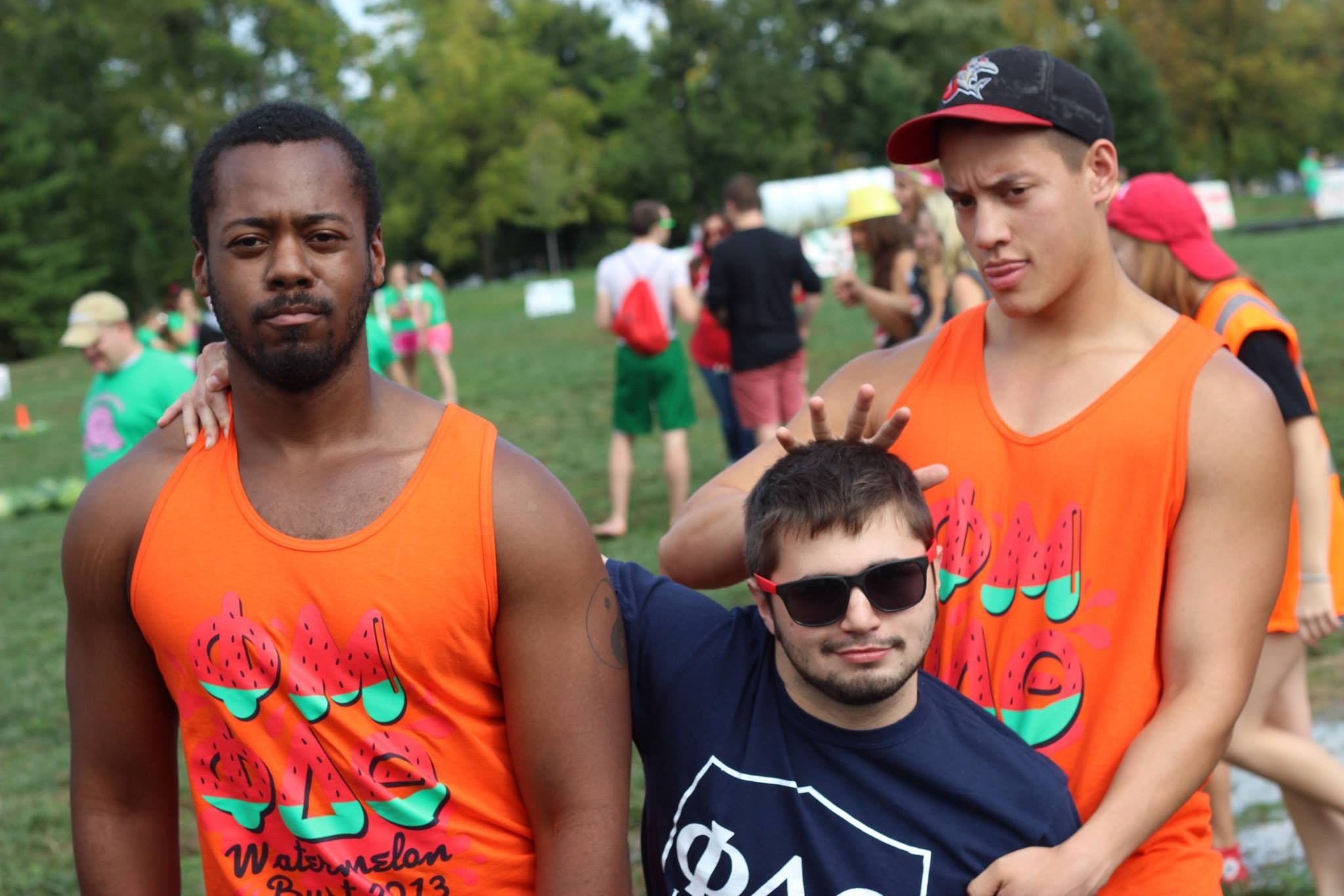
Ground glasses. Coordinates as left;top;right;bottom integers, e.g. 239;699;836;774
752;538;940;628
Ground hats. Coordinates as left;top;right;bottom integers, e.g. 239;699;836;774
836;158;944;230
885;43;1111;165
54;289;131;350
1102;170;1239;282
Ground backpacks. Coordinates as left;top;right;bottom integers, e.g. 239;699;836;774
601;246;675;357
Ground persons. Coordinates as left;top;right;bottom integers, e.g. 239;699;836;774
600;437;1084;895
364;260;458;405
58;290;197;485
1298;144;1325;216
658;43;1292;895
592;198;709;540
138;283;226;373
60;96;636;895
689;173;823;462
1106;172;1344;895
832;159;992;350
